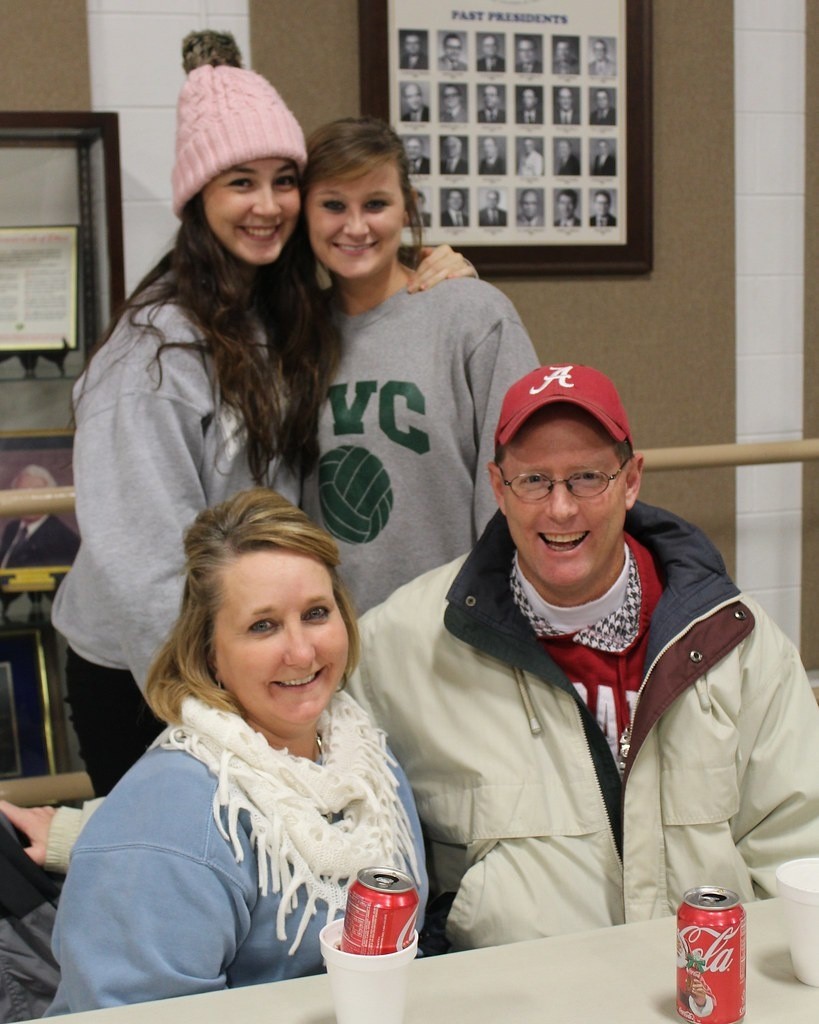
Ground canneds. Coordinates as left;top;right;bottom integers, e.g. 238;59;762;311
676;886;746;1024
340;866;419;956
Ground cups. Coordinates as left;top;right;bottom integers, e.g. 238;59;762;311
320;918;418;1024
776;858;819;988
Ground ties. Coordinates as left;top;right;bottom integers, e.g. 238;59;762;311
490;212;497;223
598;111;606;120
488;109;494;119
562;112;569;123
5;525;25;564
453;212;460;223
527;112;531;120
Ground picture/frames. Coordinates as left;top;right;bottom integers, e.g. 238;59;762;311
358;0;656;278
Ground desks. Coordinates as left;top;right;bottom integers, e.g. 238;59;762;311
0;895;819;1024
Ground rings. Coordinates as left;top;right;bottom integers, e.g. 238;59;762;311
462;259;471;267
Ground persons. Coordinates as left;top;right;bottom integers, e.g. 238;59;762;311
53;30;542;797
403;136;617;176
408;186;618;228
0;362;818;1019
399;80;615;126
0;464;79;568
400;29;616;78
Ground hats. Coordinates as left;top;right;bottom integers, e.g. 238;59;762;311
493;363;633;455
172;30;307;223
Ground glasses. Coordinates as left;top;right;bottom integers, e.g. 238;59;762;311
491;457;630;501
445;45;460;48
442;92;457;97
